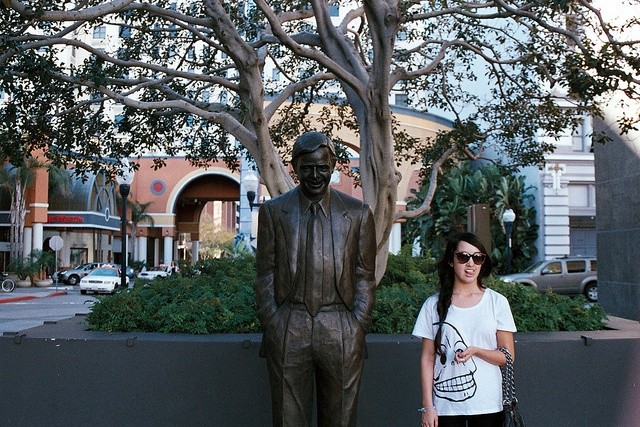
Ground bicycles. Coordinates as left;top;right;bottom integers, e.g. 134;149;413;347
0;273;15;293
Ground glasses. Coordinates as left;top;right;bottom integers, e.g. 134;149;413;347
453;251;487;265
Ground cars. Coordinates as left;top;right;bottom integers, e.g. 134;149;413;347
139;265;177;280
80;264;130;294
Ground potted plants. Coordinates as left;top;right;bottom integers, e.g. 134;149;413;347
7;249;63;287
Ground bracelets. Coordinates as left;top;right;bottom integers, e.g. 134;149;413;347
417;405;437;413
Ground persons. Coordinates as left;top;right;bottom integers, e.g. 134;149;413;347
412;233;517;427
259;131;377;427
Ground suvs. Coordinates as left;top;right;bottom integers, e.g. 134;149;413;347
497;256;599;303
58;262;118;286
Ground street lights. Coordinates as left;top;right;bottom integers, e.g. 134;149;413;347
116;158;133;287
504;209;516;274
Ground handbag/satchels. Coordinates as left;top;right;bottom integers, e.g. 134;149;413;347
495;346;526;427
